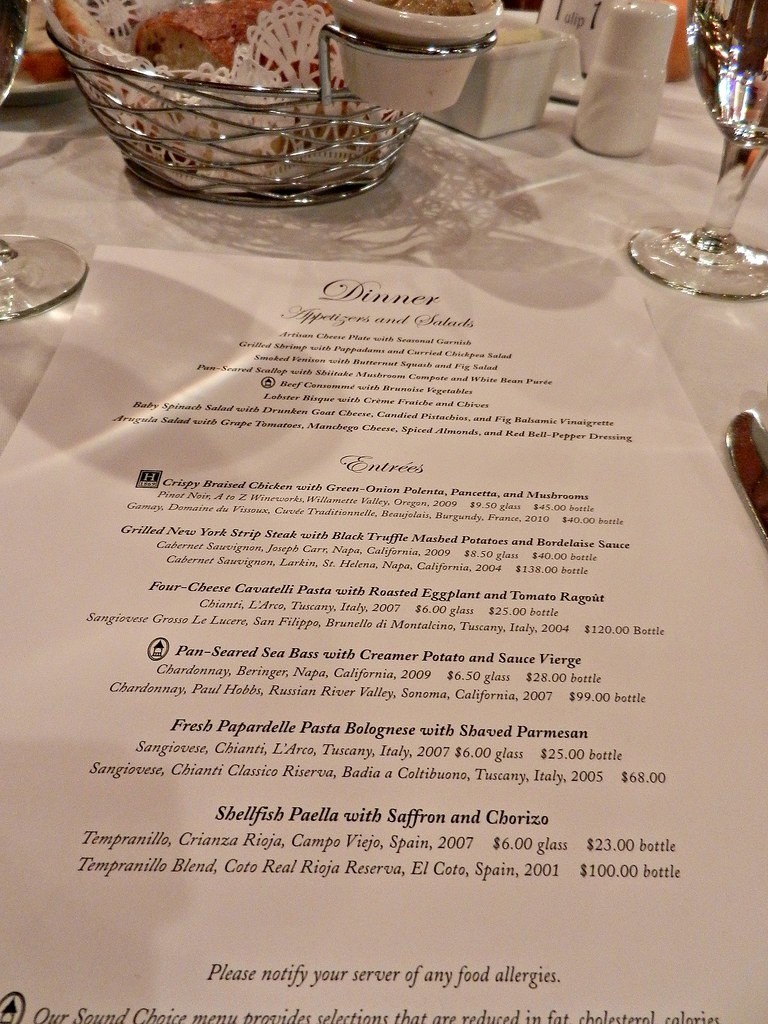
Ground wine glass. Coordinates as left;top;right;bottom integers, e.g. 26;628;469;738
624;0;768;305
0;1;89;325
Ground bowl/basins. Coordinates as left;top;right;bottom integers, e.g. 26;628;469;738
423;18;573;139
327;0;502;116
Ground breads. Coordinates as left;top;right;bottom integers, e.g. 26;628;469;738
22;0;398;167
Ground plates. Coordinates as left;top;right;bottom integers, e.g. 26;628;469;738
0;64;87;110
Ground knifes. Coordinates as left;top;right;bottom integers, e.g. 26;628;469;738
723;406;767;540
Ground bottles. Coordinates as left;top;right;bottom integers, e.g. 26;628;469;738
663;0;693;83
572;0;678;157
